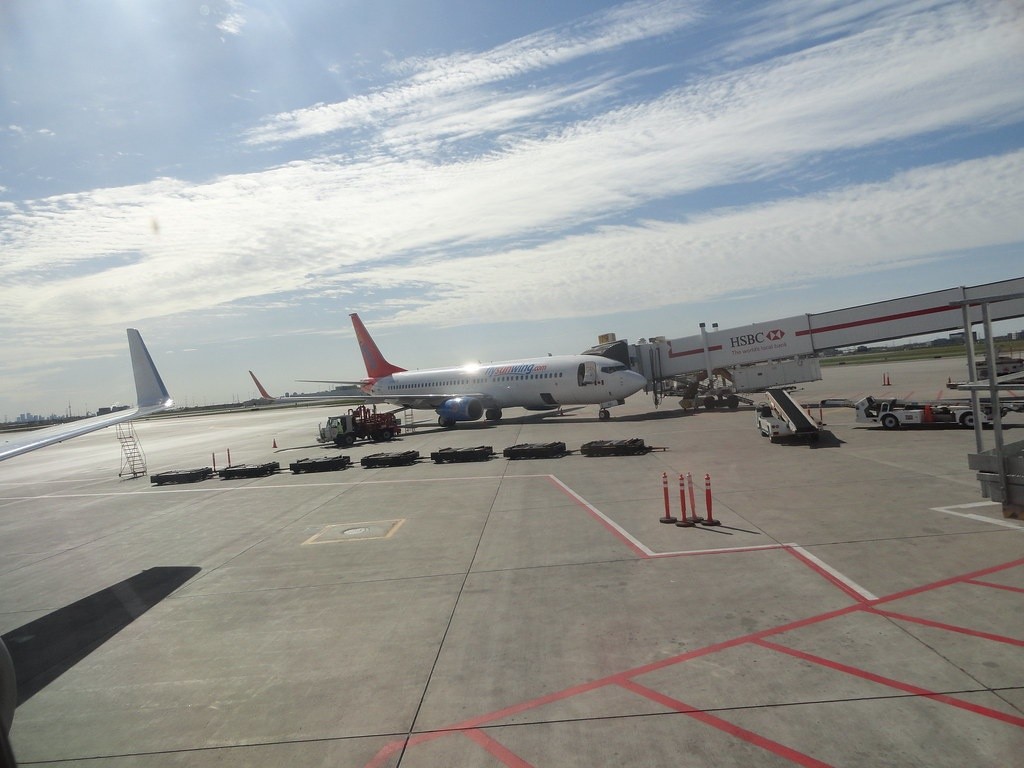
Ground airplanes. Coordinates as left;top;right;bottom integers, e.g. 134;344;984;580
249;311;649;434
0;329;173;752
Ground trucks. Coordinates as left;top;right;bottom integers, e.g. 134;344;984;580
316;406;403;446
856;394;1015;430
755;386;822;444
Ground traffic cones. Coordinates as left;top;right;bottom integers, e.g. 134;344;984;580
272;439;277;449
